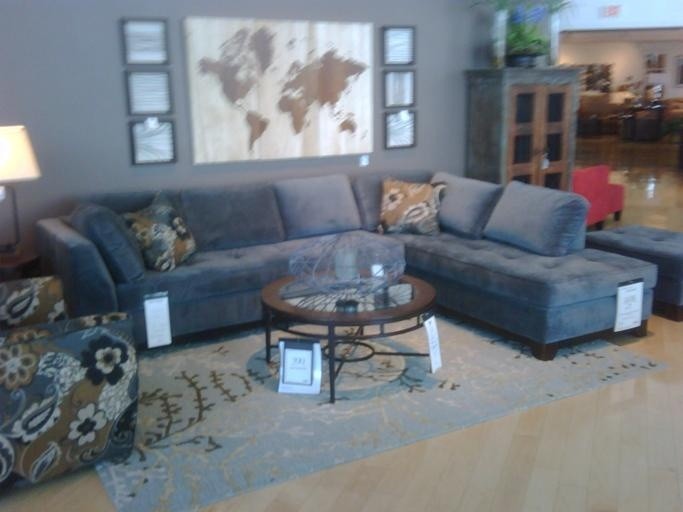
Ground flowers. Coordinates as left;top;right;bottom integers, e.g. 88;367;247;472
476;0;573;55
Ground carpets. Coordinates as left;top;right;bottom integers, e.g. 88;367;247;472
97;306;666;511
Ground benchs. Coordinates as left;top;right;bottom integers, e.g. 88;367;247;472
586;226;683;305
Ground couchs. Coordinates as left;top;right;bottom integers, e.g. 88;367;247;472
580;93;683;141
33;169;659;359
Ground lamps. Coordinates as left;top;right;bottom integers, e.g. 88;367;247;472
2;125;39;253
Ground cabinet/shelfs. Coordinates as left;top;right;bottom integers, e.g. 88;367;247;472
466;65;579;192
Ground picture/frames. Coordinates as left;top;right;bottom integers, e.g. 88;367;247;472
122;18;176;165
382;26;418;148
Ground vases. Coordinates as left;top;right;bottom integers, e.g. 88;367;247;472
510;49;538;67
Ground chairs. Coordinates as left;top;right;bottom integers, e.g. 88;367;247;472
2;276;138;486
571;164;623;229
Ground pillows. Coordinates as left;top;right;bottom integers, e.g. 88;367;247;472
121;187;197;270
378;177;447;239
